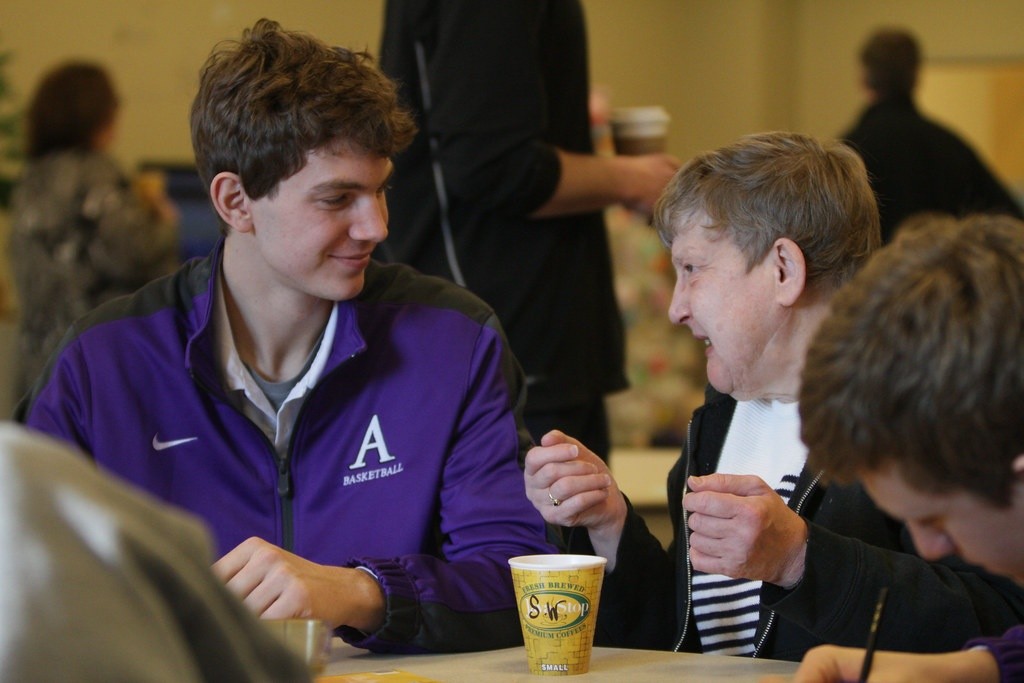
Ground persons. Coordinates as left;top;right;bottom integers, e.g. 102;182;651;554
7;61;161;406
374;0;686;554
839;27;1024;249
523;135;1024;663
792;214;1024;683
17;20;566;651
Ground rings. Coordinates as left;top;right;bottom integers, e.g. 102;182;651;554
548;489;560;506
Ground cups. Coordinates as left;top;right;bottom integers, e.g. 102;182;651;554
506;552;609;678
604;106;672;213
254;614;331;682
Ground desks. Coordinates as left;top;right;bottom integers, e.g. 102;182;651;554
607;449;682;551
312;637;804;683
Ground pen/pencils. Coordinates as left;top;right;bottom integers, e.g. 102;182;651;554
860;587;887;683
